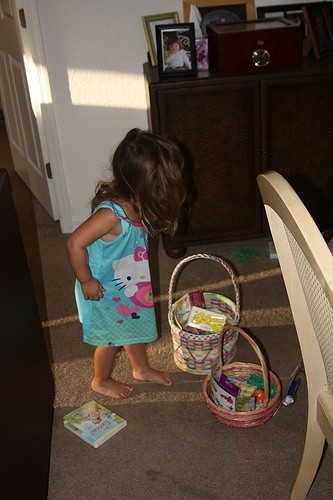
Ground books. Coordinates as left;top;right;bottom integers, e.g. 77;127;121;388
62;400;127;448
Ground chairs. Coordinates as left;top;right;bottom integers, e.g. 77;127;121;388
257;170;333;500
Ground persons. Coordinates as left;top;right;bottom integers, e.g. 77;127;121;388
66;128;187;397
164;36;192;71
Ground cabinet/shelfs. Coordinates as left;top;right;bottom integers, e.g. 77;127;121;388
135;62;333;258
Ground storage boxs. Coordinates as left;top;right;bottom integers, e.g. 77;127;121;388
207;17;302;76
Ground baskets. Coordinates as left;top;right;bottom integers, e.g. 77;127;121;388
202;325;282;429
168;254;241;376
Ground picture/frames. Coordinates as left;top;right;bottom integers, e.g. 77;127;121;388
302;1;333;59
155;22;197;76
183;0;257;39
141;11;182;66
